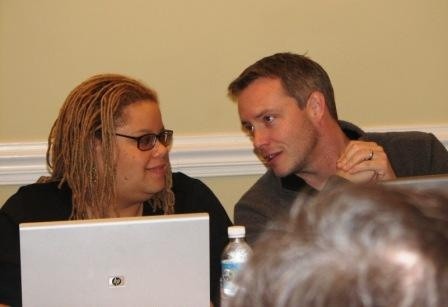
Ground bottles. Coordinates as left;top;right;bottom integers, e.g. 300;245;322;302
220;226;253;307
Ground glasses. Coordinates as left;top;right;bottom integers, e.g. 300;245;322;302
102;129;174;151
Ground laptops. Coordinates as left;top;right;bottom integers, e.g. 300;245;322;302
20;213;211;307
378;174;448;191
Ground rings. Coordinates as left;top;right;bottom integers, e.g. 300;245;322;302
369;150;374;159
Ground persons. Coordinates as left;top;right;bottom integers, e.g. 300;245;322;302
0;73;234;307
234;182;448;306
227;52;448;250
